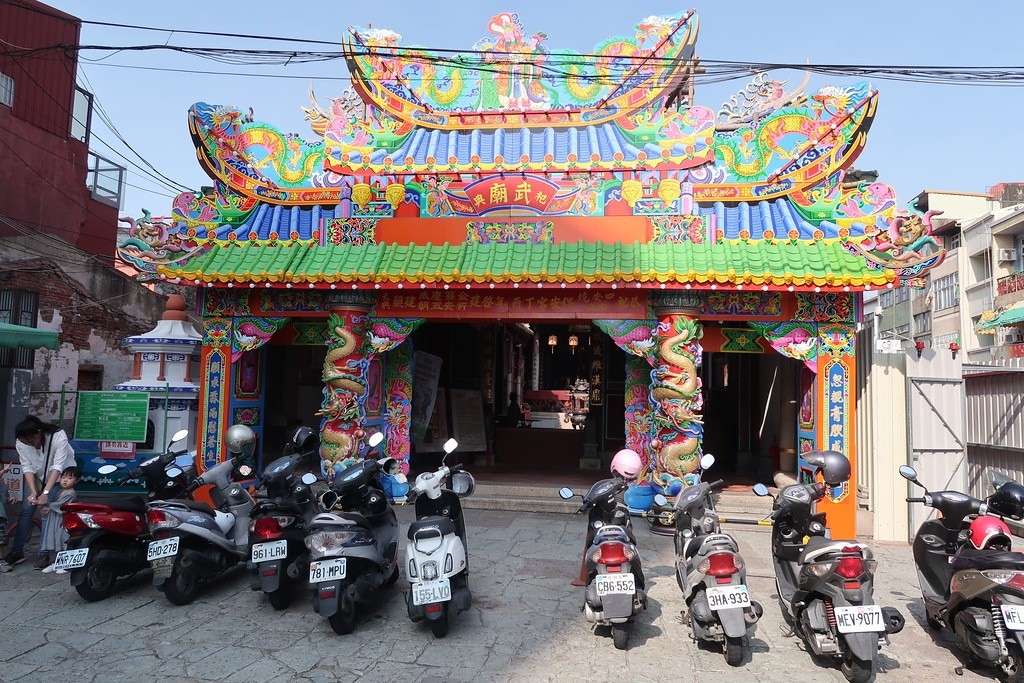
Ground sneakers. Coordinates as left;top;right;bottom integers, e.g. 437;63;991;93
0;560;13;572
4;551;25;566
33;554;49;569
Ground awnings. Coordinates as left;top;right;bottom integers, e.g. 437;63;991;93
974;307;1024;334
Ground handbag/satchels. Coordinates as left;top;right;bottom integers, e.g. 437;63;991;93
34;475;43;500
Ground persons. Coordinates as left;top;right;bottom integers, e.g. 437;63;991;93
0;416;76;572
0;461;17;558
41;466;76;574
506;392;520;428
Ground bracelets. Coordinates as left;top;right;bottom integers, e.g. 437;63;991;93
43;490;50;495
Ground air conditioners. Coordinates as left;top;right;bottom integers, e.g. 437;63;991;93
874;306;883;316
999;248;1017;261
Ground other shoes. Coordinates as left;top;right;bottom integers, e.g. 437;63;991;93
56;569;68;574
42;563;57;573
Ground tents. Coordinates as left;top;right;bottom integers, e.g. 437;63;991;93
0;322;58;350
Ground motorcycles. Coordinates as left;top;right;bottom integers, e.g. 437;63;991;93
558;477;649;651
898;463;1024;683
390;438;474;637
239;442;316;611
147;448;262;605
301;432;401;634
752;465;906;683
655;453;764;666
58;428;191;602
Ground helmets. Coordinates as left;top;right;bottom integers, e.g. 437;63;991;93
988;482;1024;521
452;470;475;498
214;509;236;535
290;426;318;448
821;451;851;485
610;449;642;479
967;515;1012;551
224;424;256;454
